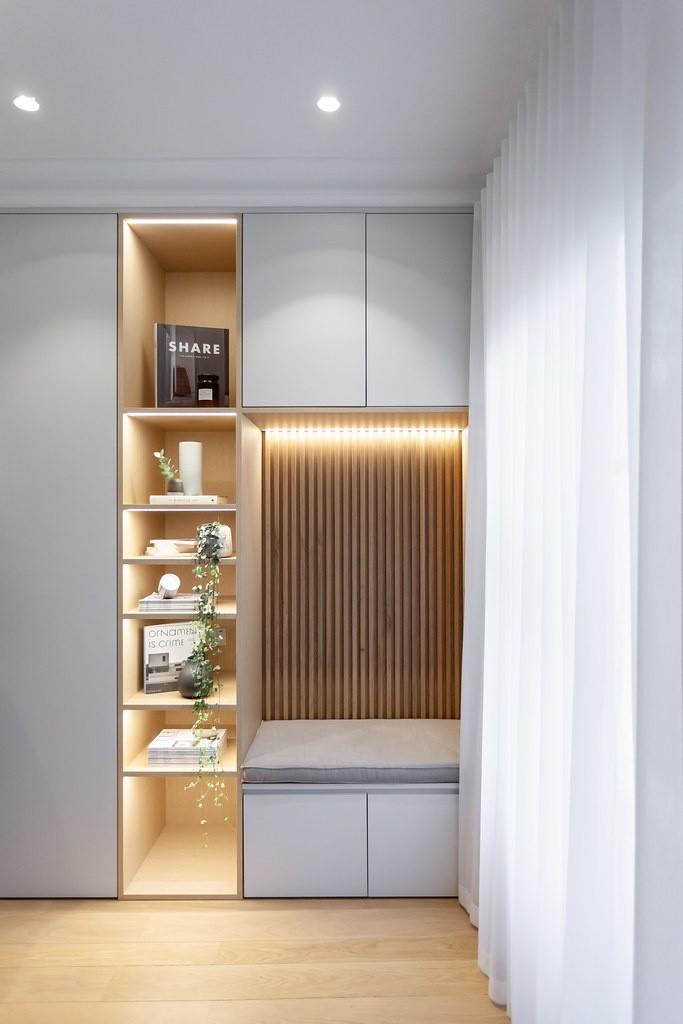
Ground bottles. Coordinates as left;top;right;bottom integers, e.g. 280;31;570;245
178;655;214;698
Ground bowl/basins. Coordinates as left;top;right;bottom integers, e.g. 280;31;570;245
150;538;197;553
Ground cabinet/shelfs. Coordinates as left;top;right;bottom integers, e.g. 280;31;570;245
119;408;244;899
242;213;468;406
243;786;460;897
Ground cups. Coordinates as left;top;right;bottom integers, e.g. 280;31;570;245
157;573;180;599
177;441;203;495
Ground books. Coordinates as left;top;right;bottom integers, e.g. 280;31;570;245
144;538;199;558
143;622;205;695
148;729;226;768
139;592;215;613
148;494;229;505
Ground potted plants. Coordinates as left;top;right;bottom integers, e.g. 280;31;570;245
185;518;234;846
153;449;182;495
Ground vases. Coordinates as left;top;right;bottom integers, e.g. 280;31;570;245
179;655;212;698
